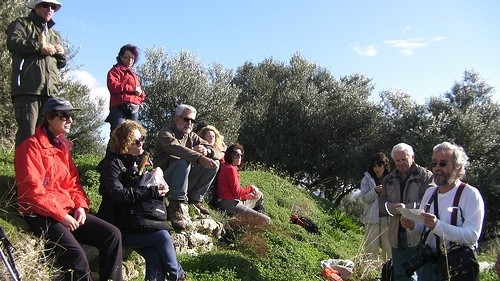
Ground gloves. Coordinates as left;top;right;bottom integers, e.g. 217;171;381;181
135;86;142;96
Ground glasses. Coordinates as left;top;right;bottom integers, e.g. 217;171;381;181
371;160;384;166
136;136;146;147
37;2;56;9
431;159;448;167
235;153;244;157
55;112;74;121
178;115;197;124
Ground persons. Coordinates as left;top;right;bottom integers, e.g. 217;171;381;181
97;120;186;281
378;143;437;281
198;125;226;152
217;143;272;225
13;98;122;281
360;153;391;260
6;0;66;150
400;142;484;281
154;104;220;230
106;44;144;132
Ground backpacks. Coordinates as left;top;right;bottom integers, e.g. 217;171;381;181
113;168;169;232
381;257;395;281
290;214;321;236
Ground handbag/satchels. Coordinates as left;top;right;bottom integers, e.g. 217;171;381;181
441;243;480;281
121;102;139;114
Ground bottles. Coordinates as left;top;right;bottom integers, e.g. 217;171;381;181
38;31;46;43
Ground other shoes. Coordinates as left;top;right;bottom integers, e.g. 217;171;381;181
188;196;210;214
168;199;186;230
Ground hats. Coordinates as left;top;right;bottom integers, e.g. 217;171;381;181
26;0;62;12
40;97;83;115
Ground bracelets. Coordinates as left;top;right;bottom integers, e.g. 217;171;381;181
206;149;212;158
